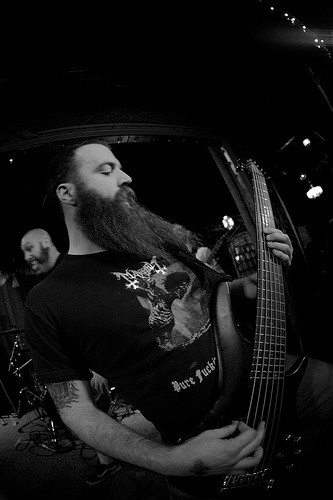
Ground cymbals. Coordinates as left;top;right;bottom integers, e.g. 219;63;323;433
0;327;24;334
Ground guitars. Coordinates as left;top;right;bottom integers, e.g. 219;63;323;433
156;154;310;500
205;225;237;265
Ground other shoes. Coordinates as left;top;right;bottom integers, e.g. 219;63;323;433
85;461;119;485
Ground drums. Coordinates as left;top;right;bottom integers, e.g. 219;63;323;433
40;385;66;428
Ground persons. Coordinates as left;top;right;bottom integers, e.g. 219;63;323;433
21;228;121;485
22;139;333;500
